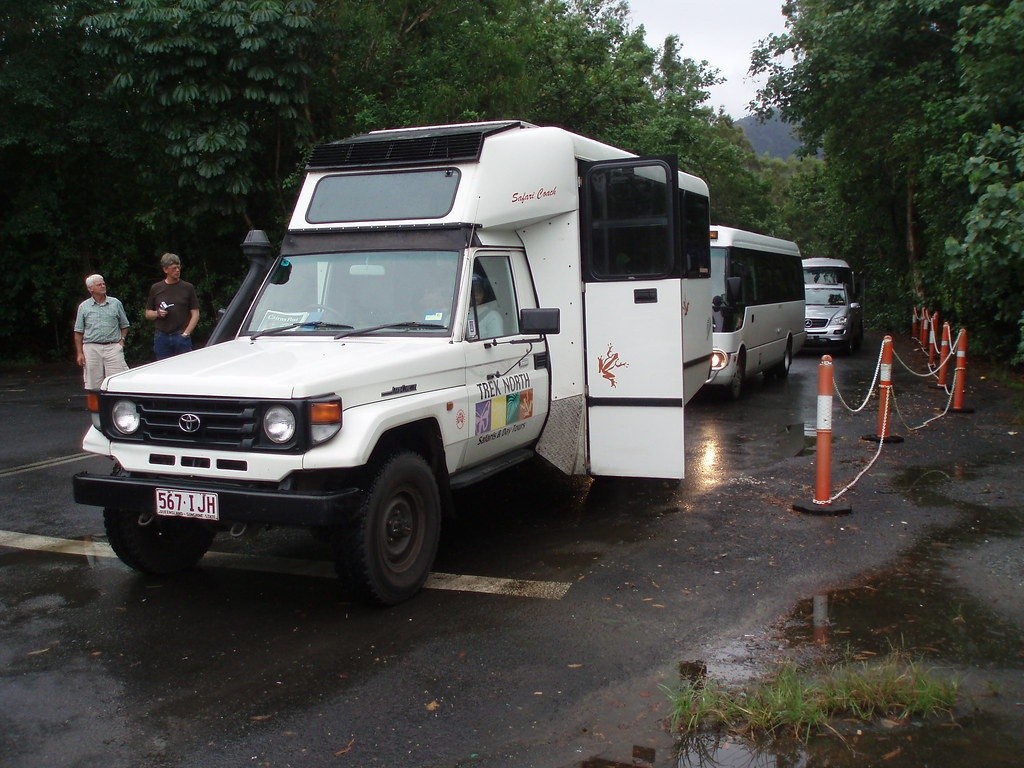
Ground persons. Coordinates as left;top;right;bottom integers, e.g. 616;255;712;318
144;253;201;361
73;274;130;430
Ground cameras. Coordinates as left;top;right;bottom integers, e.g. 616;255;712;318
160;301;175;311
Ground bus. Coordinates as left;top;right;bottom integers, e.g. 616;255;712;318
803;256;857;300
708;226;808;397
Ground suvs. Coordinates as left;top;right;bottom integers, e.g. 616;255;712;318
804;283;862;355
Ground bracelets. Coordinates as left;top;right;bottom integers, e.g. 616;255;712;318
182;331;191;338
121;337;126;342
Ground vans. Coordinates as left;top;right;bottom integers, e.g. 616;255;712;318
73;122;708;612
